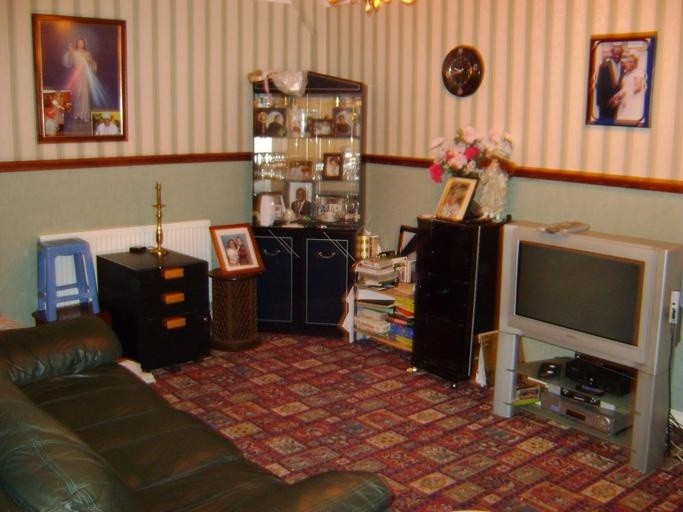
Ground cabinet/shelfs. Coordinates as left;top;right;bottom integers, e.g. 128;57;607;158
96;247;209;373
492;330;670;474
413;213;510;388
353;256;413;352
252;68;364;338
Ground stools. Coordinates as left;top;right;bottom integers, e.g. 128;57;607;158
36;237;101;321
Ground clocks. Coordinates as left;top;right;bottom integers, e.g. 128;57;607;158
442;45;482;98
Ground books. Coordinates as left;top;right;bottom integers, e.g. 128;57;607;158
472;327;525;389
339;251;415;353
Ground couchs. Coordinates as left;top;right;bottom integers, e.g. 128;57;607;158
0;318;397;510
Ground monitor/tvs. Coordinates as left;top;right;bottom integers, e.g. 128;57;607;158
498;220;683;376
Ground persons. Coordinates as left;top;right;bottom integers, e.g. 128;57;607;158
225;239;238;266
57;37;100;123
235;236;251;266
93;113;119;136
325;158;339;179
336;115;350;135
439;190;459;216
609;54;643;120
290;186;310;220
262;112;284;138
594;44;625;119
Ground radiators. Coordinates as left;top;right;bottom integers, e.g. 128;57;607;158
40;220;211;309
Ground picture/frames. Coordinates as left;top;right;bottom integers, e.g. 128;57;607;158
209;224;265;276
585;32;655;129
33;14;129;141
432;176;478;223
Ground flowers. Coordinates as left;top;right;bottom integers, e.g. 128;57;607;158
428;126;489;184
